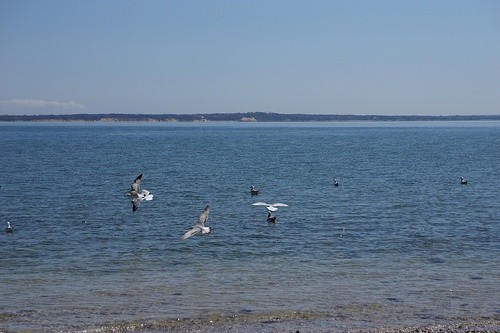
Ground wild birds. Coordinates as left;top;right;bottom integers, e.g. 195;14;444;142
267;211;277;224
249;185;260;195
334;179;338;186
178;205;212;240
125;173;154;213
5;222;14;233
252;201;289;212
461;176;467;184
